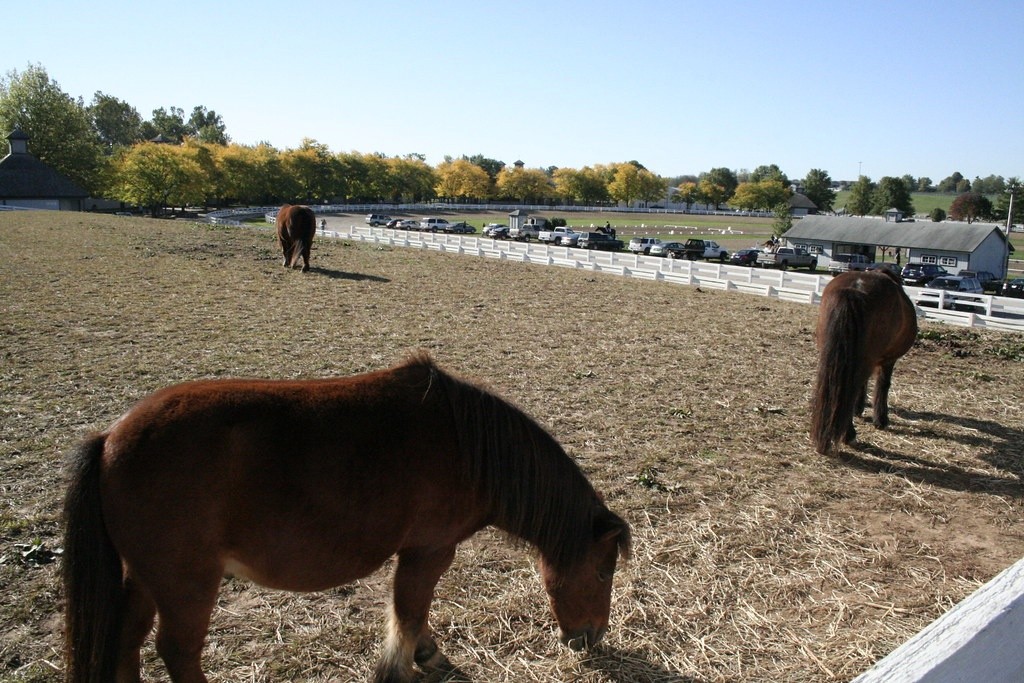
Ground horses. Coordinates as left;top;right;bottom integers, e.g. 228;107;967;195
61;350;632;683
810;263;917;452
276;204;316;274
761;240;774;248
595;226;616;239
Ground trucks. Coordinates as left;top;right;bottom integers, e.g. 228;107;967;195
667;239;729;264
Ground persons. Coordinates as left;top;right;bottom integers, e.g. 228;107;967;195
667;250;674;259
320;219;326;231
606;221;611;232
462;220;467;233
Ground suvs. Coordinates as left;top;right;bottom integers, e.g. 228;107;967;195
901;262;956;286
366;214;392;227
628;238;662;255
829;254;873;277
421;218;449;233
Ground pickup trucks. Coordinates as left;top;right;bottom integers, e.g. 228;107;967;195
756;247;818;272
510;224;542;242
538;227;574;246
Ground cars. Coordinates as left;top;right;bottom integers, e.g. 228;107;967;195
446;222;477;234
917;269;1024;311
386;218;421;231
649;242;685;256
483;224;510;240
561;233;625;252
865;262;903;275
730;249;764;267
